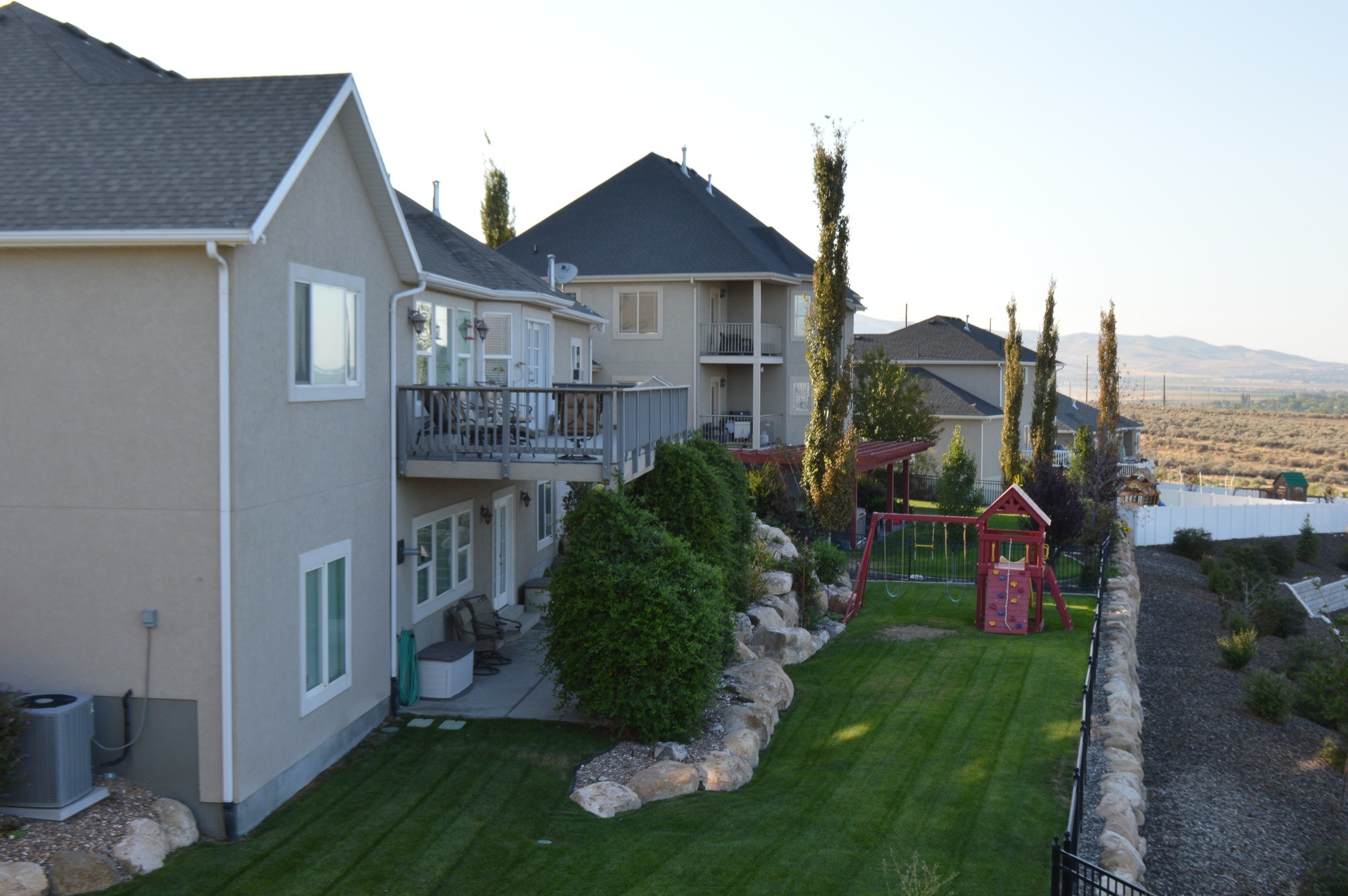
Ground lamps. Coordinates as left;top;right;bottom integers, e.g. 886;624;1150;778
475;318;488;343
721;377;725;388
523;492;531;508
481;505;493;525
409;308;428;339
459;317;469;343
398;539;430;565
721;287;726;298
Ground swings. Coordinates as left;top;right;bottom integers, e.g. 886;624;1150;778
942;522;969;604
880;520;909;599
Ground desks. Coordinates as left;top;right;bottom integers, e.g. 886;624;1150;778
526;577;553;614
414;640;477;700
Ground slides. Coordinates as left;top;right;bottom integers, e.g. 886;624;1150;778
999;555;1036;607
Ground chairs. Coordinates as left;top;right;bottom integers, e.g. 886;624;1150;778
703;409;774;444
548;387;605;460
409;378;540;460
719;333;751;355
446;594;522;677
